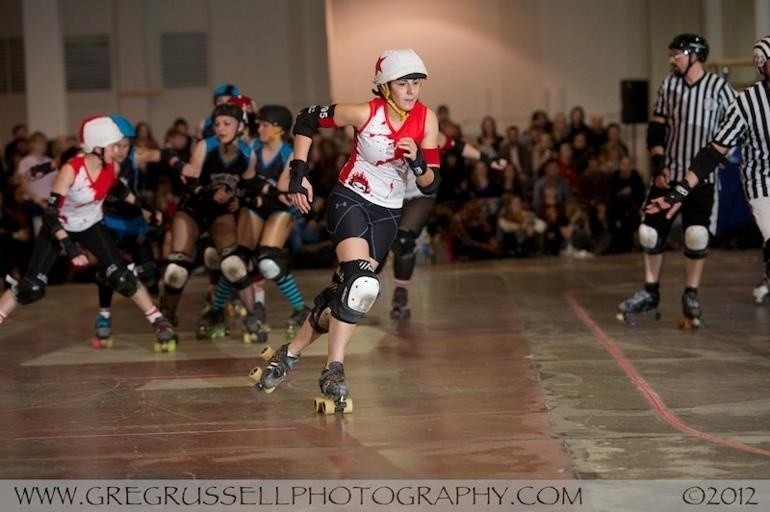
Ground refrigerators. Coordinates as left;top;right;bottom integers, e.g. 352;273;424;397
153;302;310;351
390;287;410;319
678;293;702;329
91;313;114;348
616;290;660;323
249;342;299;393
752;278;769;304
314;362;353;414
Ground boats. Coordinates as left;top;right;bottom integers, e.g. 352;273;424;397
619;79;649;125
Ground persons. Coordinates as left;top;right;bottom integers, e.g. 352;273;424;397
261;45;444;397
640;34;770;299
616;31;742;321
1;79;685;348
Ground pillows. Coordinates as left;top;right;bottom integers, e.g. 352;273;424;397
374;49;428;84
669;33;708;62
80;114;135;153
213;84;257;121
753;35;770;73
258;104;293;133
